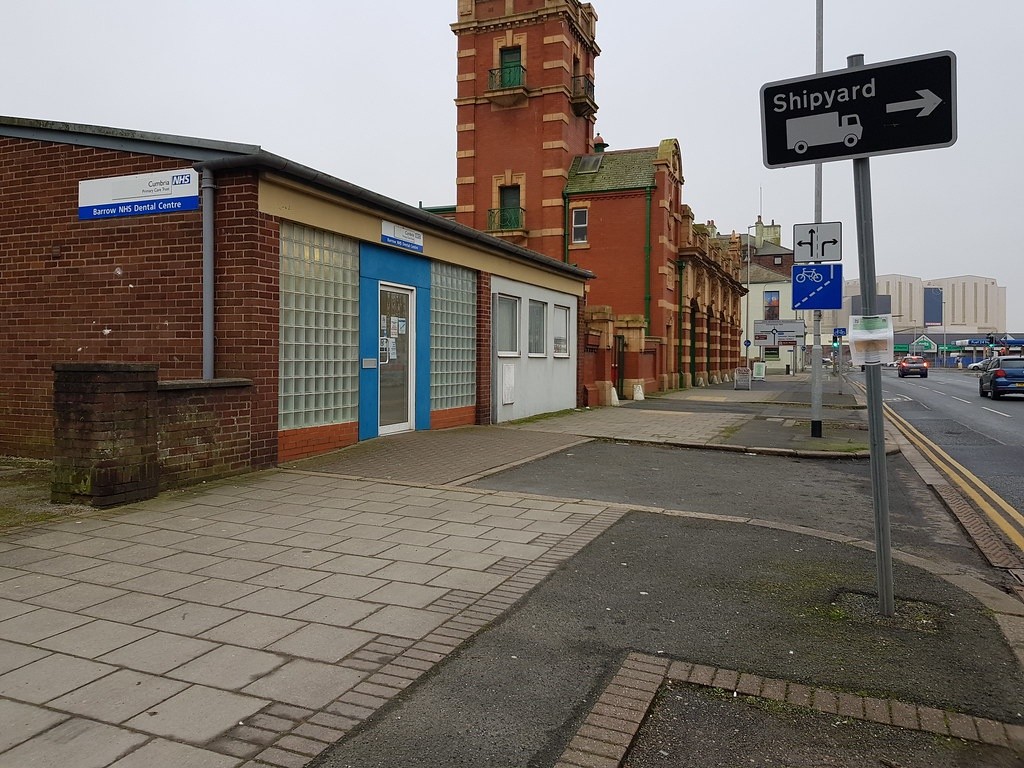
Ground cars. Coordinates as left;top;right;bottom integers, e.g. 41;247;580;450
887;360;901;367
896;356;927;378
978;355;1024;400
968;358;992;371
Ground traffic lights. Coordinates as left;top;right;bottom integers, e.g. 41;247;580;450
833;336;838;347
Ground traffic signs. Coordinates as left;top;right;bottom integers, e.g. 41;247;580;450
759;48;958;170
